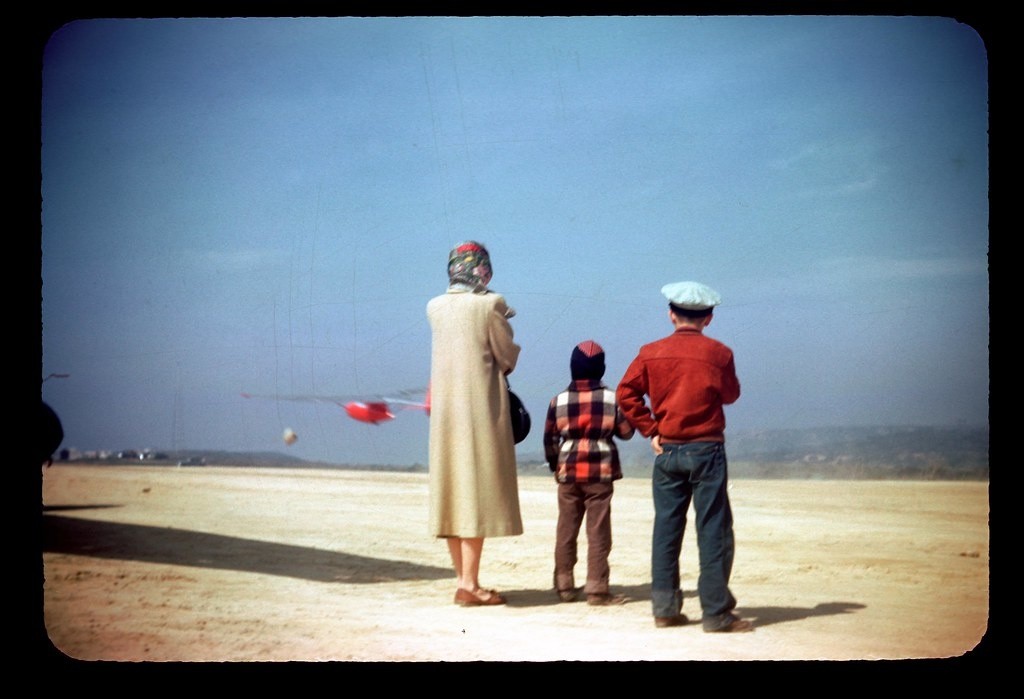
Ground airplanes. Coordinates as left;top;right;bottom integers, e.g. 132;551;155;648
240;386;424;426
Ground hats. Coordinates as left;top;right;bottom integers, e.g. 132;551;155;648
571;340;605;379
661;282;722;318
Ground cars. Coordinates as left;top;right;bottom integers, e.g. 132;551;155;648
179;457;206;467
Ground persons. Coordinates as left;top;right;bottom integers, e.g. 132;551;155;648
426;240;531;608
616;278;750;632
543;338;639;607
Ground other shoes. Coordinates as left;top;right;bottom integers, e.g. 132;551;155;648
703;617;751;632
655;613;689;628
454;589;508;606
558;587;576;602
587;592;623;605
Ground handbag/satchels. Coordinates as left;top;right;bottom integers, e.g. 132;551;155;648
508;390;531;444
